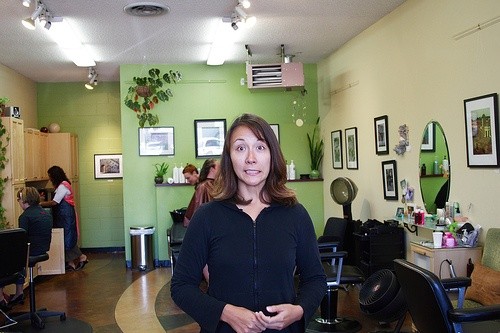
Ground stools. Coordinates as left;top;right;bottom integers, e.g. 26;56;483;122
5;253;66;330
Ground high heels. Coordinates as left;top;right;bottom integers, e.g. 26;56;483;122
8;294;25;305
0;299;7;309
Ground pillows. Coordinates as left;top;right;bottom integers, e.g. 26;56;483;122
465;263;500;305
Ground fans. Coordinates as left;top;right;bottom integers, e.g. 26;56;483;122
357;269;406;329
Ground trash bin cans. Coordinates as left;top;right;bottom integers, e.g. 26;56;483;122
129;226;155;272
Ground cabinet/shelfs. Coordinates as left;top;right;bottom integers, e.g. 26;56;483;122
353;228;405;277
409;240;483;300
0;117;81;296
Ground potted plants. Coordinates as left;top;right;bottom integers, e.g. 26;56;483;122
306;116;324;180
154;162;169;184
124;68;182;128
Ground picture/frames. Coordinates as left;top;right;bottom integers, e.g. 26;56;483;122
194;119;227;158
420;122;436;152
94;154;123;179
270;124;280;145
396;208;404;217
138;127;175;156
464;93;500;168
331;115;399;201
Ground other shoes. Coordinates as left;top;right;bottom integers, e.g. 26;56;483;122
65;265;74;271
76;258;88;270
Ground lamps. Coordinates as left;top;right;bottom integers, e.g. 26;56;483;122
85;67;98;90
22;0;51;30
231;5;248;30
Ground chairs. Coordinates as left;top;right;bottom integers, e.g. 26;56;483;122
0;228;28;328
392;228;500;333
292;241;364;324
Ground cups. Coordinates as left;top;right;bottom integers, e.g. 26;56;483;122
415;212;424;225
432;232;443;248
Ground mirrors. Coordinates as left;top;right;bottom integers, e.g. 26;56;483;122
418;121;451;215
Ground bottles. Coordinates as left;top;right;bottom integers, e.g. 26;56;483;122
40;127;48;133
49;122;61;133
168;161;201;184
285;160;295;180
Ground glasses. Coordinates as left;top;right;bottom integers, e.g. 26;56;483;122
17;198;21;202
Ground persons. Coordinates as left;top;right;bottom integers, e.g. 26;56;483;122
170;113;326;333
196;159;221;284
0;187;52;311
182;164;199;227
39;166;88;272
